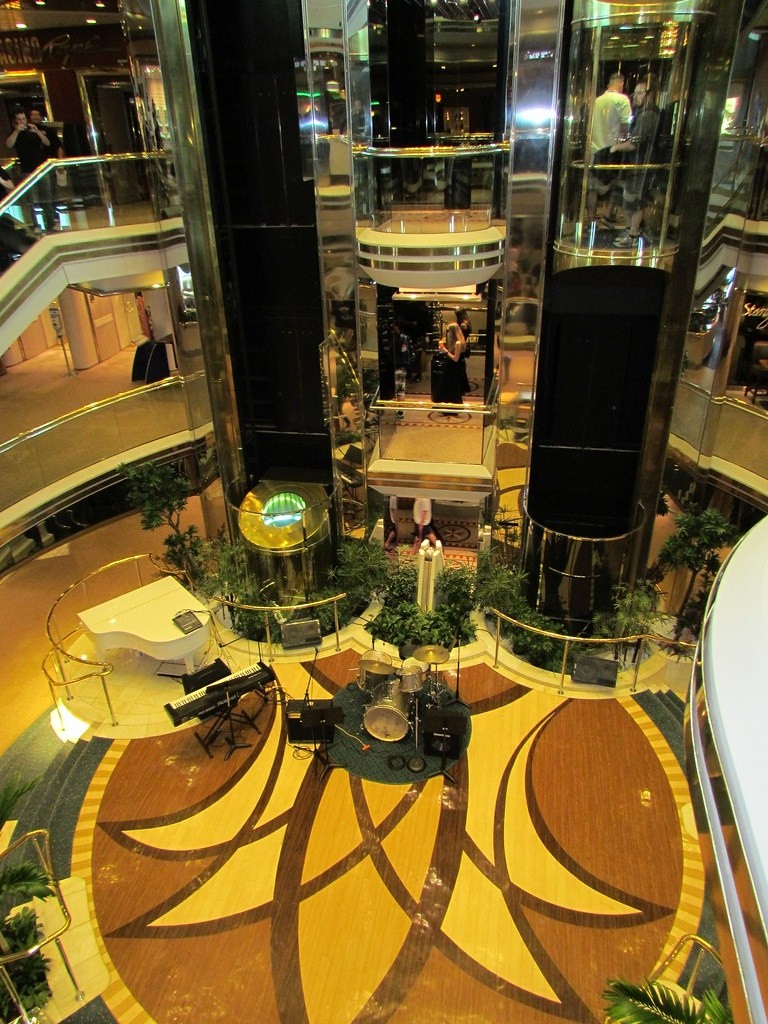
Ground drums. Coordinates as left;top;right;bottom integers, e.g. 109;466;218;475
401;658;429;682
356;666;389;693
362;650;392;664
363;680;411;742
395;665;422;692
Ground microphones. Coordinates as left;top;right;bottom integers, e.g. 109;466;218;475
315;646;319;652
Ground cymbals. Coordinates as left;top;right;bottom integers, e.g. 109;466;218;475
414;645;450;664
358;659;394;674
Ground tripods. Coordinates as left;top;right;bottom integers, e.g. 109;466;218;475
293;651;346;781
205;619;290;761
433;611;472;709
422;720;465;784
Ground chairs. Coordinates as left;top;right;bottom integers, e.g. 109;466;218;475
181;657;263;758
743;341;768;404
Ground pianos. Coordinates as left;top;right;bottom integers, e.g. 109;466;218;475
163;661;279;726
75;573;212;674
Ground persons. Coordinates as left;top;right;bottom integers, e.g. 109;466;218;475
608;81;661;253
441;321;472;415
586;71;633;234
454;305;472;340
29;106;65;222
392;326;411;418
5;105;56;232
384;495;398;553
410;496;441;557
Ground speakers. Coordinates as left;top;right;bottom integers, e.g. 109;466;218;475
285;700;333;744
571;654;619;687
422;709;468;760
281;619;322;649
181;658;238;720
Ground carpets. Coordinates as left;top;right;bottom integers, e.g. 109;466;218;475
324;671;473;785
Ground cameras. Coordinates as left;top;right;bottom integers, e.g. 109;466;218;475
21;125;28;130
